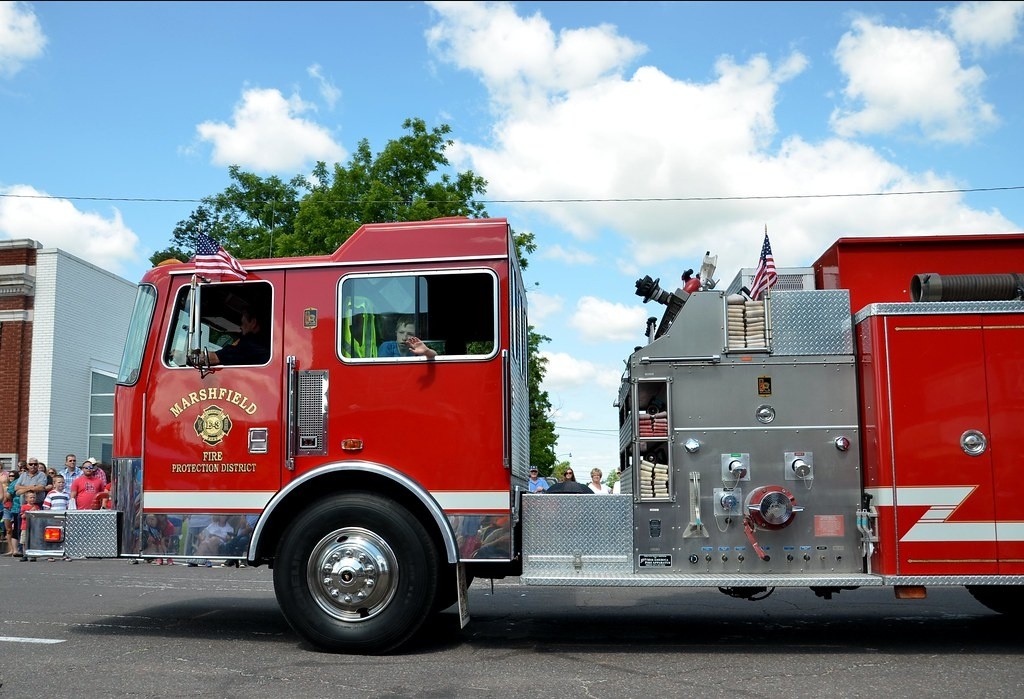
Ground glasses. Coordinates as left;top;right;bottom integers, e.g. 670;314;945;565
69;460;77;463
9;475;15;477
617;472;620;474
29;463;38;466
566;472;572;474
83;466;94;469
530;471;537;473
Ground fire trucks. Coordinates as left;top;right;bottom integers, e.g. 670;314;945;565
19;213;1024;658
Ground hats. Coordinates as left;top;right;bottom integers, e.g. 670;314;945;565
530;466;538;471
88;458;97;464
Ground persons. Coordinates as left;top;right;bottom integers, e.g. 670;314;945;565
563;468;576;482
529;465;549;494
125;557;251;568
587;468;612;494
379;315;438;358
0;455;112;562
749;235;777;300
111;458;260;558
167;306;271;365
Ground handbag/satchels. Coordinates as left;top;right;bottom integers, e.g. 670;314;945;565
68;497;76;510
4;499;12;509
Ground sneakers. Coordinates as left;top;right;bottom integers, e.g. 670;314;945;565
156;559;163;566
167;558;173;566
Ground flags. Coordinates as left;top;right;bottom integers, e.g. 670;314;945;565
194;230;247;283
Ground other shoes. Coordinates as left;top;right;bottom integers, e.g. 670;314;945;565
1;550;23;557
143;559;153;564
128;560;138;565
20;557;28;562
30;557;36;561
80;556;87;559
188;561;246;568
63;556;72;561
48;558;55;561
